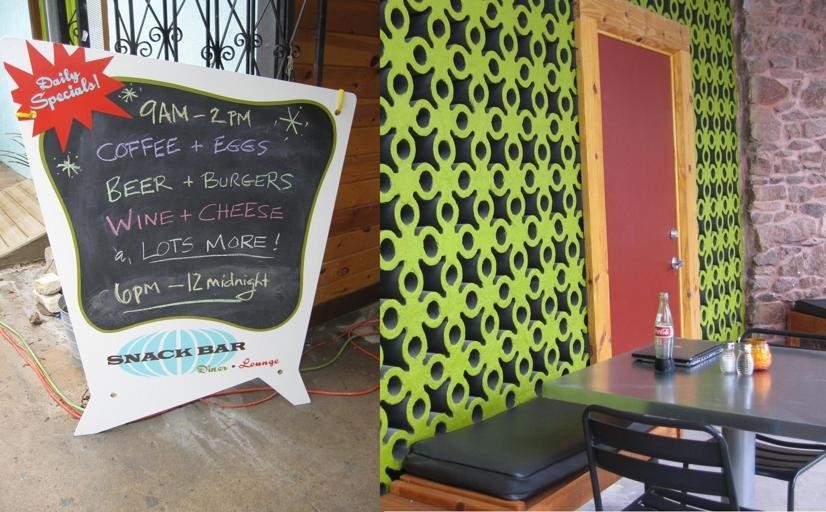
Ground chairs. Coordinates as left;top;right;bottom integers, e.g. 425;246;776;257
754;432;826;512
582;403;757;511
705;329;826;511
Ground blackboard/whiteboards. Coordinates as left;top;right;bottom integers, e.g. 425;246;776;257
24;74;355;350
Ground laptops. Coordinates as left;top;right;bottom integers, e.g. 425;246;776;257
631;337;723;367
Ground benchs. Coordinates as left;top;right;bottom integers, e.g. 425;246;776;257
380;396;685;511
786;296;826;348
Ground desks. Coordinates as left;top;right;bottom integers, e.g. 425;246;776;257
541;340;826;511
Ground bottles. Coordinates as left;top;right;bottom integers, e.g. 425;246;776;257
719;338;773;377
654;293;675;373
